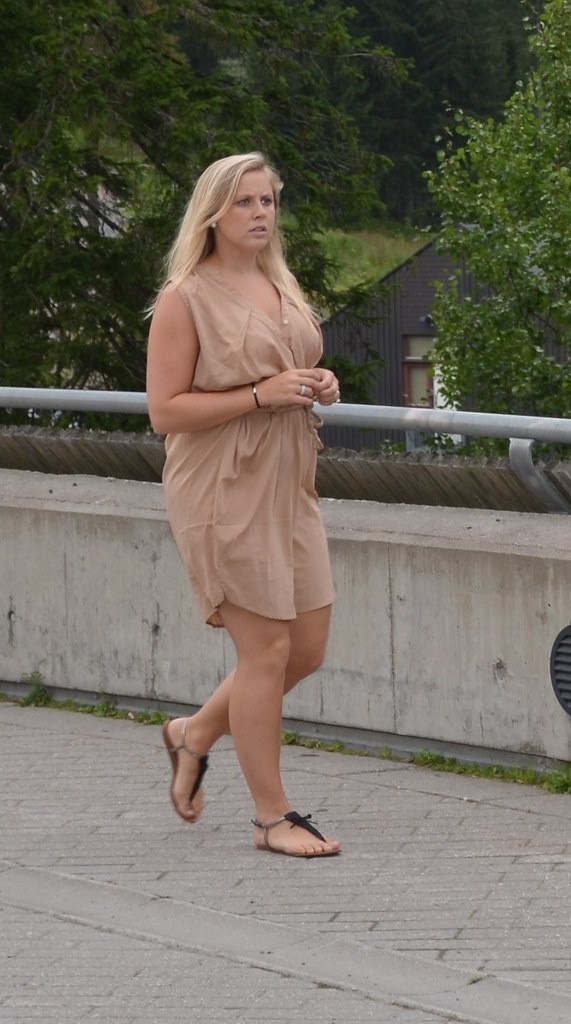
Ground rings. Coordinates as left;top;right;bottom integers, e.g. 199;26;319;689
300;385;306;395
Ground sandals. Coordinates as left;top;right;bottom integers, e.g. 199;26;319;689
249;808;342;858
161;717;210;824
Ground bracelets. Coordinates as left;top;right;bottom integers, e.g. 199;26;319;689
252;383;260;408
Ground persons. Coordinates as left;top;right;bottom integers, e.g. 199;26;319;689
146;152;340;857
